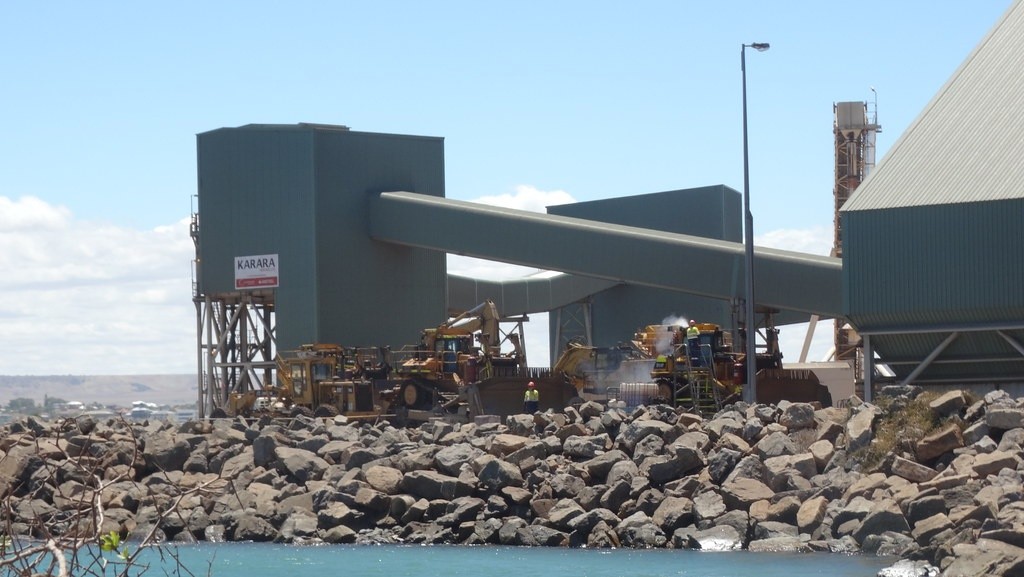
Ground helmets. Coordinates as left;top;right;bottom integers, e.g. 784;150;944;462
528;382;534;387
689;320;695;324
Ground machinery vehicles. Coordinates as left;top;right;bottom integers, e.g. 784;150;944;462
729;307;834;409
536;318;742;411
227;344;396;425
393;301;527;410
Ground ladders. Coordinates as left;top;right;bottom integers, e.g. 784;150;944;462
673;343;723;417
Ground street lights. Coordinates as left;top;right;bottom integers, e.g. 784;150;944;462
741;42;772;404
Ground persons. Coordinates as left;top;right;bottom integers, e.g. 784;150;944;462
524;382;539;415
682;320;701;360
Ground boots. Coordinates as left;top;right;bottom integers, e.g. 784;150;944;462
690;357;699;367
